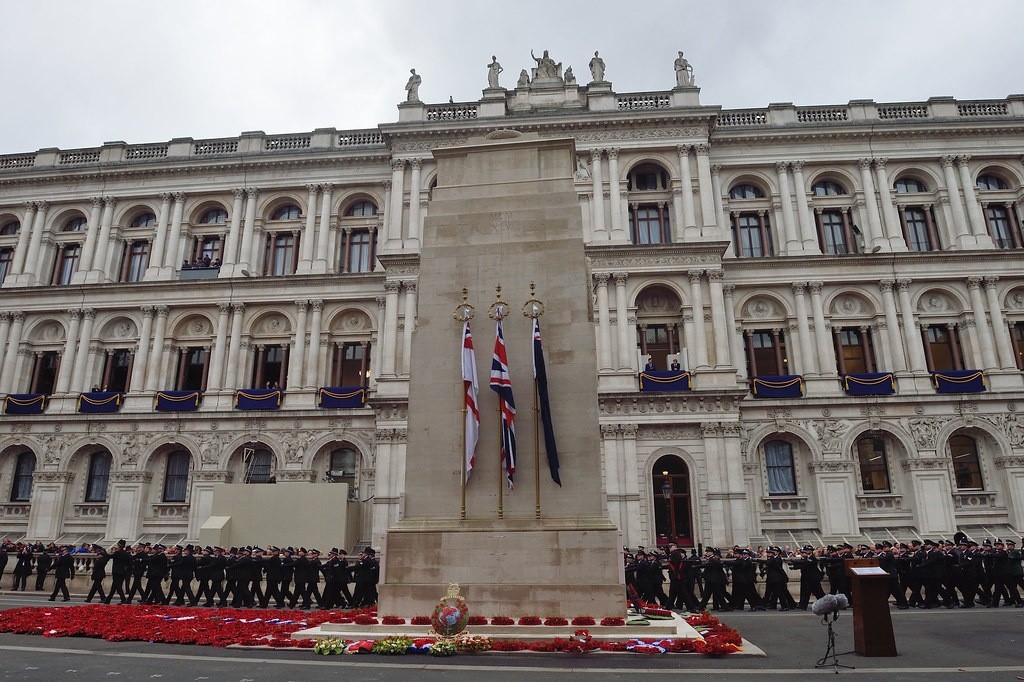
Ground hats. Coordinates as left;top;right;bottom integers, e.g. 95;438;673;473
63;546;69;550
42;549;48;552
96;543;376;558
623;536;1024;559
692;565;695;568
117;540;126;548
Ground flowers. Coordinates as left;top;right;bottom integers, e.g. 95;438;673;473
0;603;743;656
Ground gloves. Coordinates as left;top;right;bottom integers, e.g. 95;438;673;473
751;559;755;562
788;563;791;566
46;568;49;571
91;574;95;581
789;567;793;570
761;568;764;572
71;576;74;579
763;570;766;573
164;576;168;582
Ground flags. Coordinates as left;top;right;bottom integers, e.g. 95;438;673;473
461;309;480;486
489;306;517;489
532;303;562;488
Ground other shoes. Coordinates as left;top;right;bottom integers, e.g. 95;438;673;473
61;599;70;601
84;600;366;609
666;602;853;612
48;598;55;601
87;570;91;572
11;588;17;591
892;599;1024;609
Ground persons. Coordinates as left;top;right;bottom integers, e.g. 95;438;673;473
531;48;555;78
181;254;222;267
624;532;1024;611
0;538;380;609
405;68;422;101
264;382;279;389
674;51;692;85
589;50;606;82
645;358;655;371
671;359;680;370
92;383;109;392
487;54;504;87
